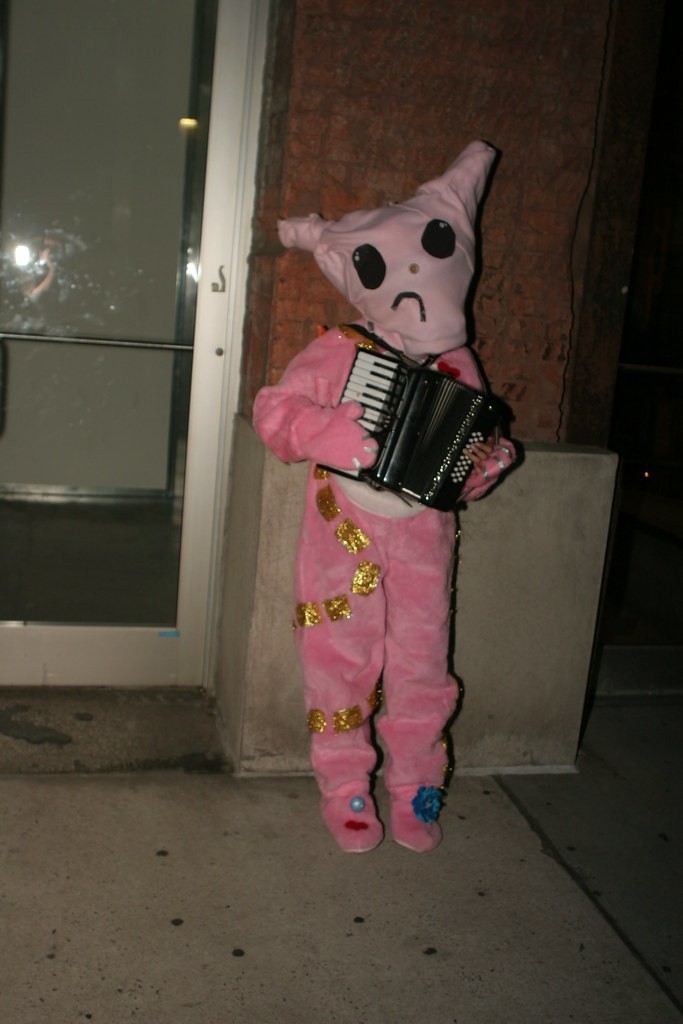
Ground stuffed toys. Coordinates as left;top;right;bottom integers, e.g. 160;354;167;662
252;140;518;852
